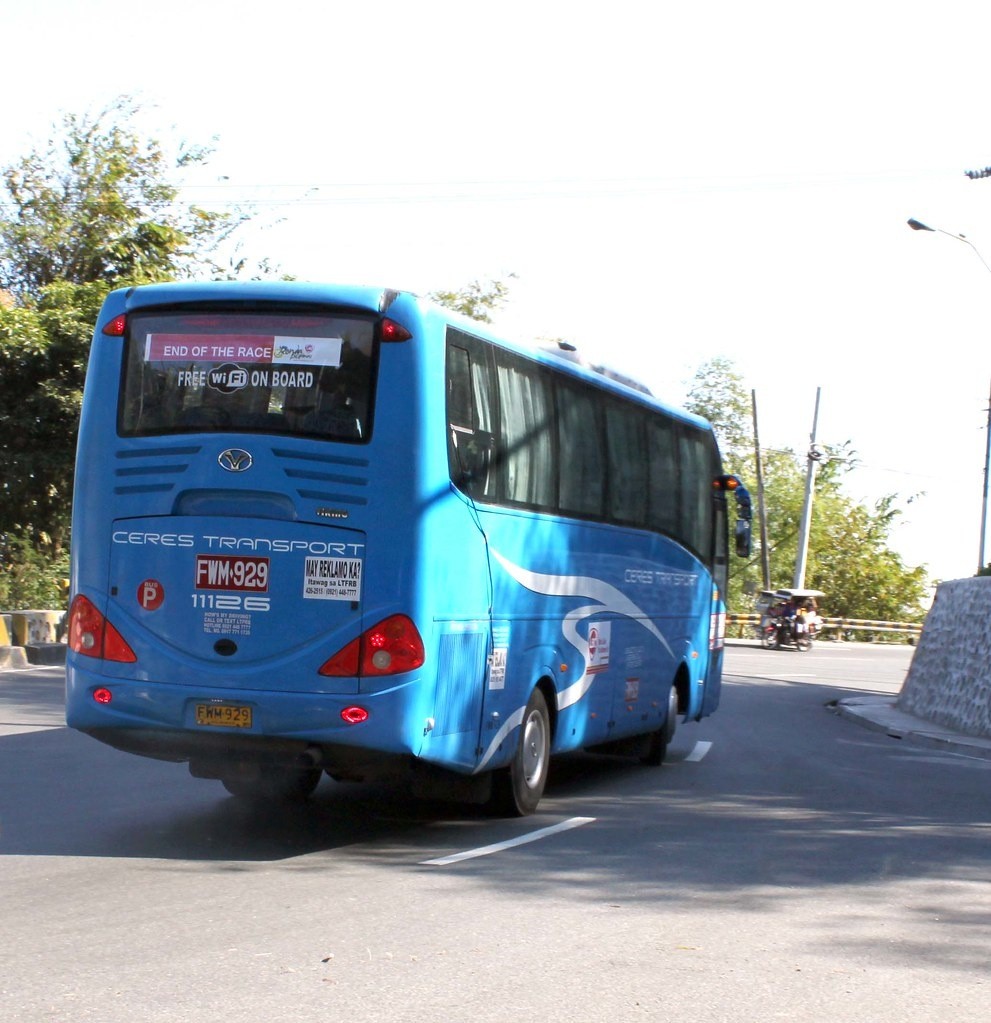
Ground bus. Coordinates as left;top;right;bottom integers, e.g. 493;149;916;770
67;282;752;819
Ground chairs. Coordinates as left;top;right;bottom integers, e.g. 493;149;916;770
173;404;365;442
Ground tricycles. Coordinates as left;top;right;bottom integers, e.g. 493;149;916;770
756;587;827;651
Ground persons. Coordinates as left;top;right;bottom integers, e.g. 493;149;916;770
132;373;183;415
330;391;353;413
783;597;798;639
